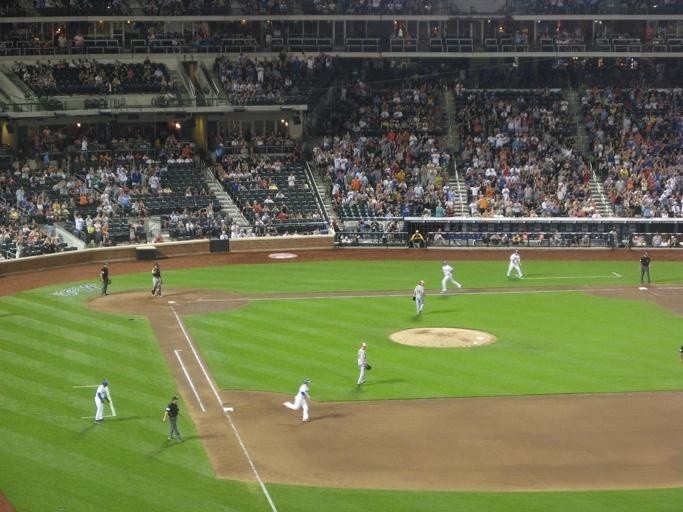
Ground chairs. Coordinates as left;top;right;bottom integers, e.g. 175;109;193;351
0;0;683;260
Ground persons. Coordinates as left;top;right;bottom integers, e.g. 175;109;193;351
639;250;651;283
93;379;110;420
2;2;682;259
355;342;371;387
505;247;524;279
99;261;110;296
150;261;163;298
412;279;425;316
280;377;312;422
162;395;185;442
440;260;463;293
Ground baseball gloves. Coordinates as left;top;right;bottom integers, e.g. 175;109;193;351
365;364;372;371
413;297;416;301
107;280;111;284
